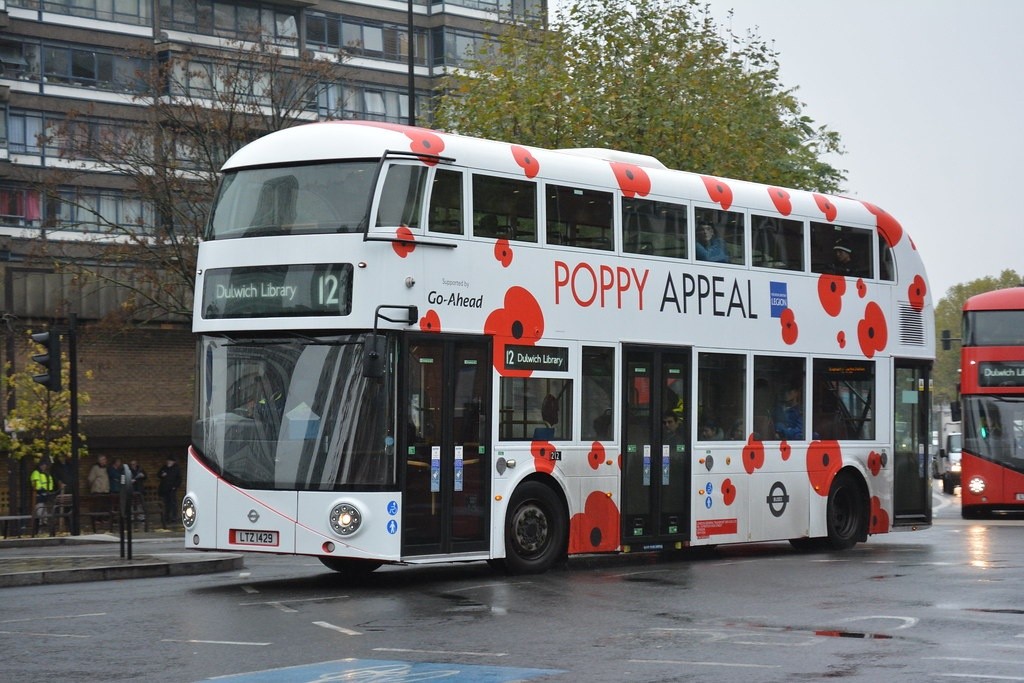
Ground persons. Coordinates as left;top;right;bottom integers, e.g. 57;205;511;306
662;411;685;461
687;217;728;262
667;385;683;415
123;460;147;521
31;458;57;538
833;242;862;274
155;456;182;524
772;382;819;439
87;454;110;524
699;413;732;440
108;456;122;526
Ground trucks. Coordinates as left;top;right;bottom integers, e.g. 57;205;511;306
940;409;969;495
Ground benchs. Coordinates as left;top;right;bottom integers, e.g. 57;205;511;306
406;219;870;277
0;489;168;539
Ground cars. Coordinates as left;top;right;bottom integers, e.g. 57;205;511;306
941;285;1024;520
931;451;944;479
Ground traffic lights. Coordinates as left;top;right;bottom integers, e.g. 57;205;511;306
30;323;63;394
978;413;990;439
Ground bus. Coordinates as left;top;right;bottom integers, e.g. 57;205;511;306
176;122;939;578
